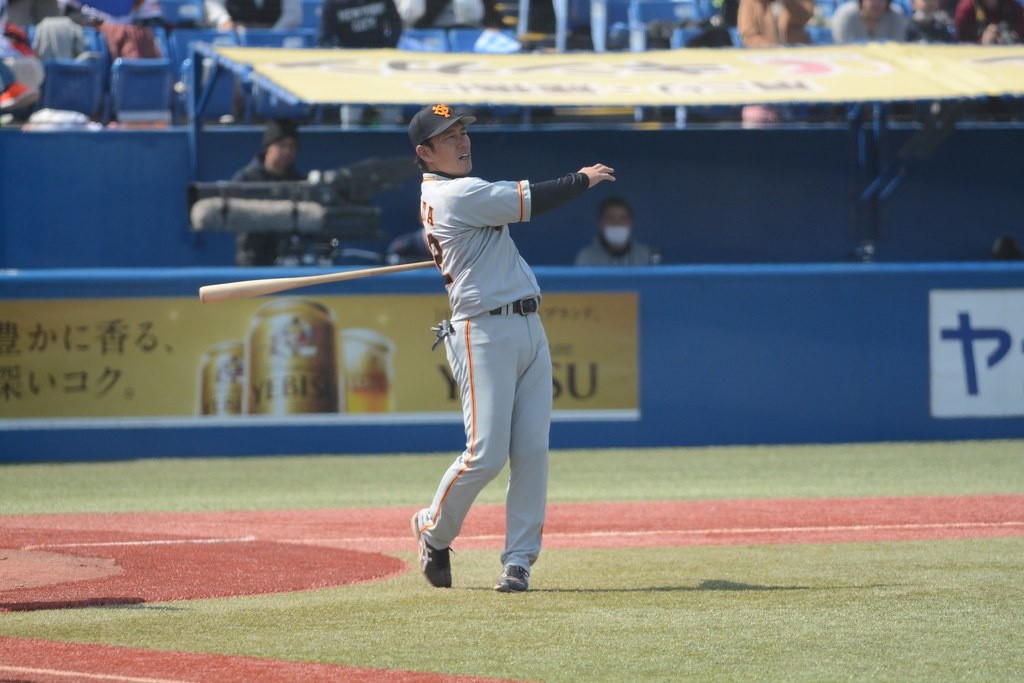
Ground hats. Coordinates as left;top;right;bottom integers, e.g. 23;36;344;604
261;118;300;148
408;103;477;151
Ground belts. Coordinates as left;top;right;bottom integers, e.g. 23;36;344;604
490;296;540;316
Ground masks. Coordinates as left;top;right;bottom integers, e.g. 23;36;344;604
603;226;631;247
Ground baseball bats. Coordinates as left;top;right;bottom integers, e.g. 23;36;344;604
197;258;436;305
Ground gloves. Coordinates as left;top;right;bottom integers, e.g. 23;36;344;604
430;320;455;351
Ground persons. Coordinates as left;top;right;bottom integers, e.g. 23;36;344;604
0;0;488;123
675;0;1024;125
578;199;652;266
408;103;616;593
230;119;325;267
389;224;431;266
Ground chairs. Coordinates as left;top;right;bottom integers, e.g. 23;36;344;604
0;0;957;125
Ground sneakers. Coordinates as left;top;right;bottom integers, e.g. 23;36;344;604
411;511;456;588
494;565;529;593
